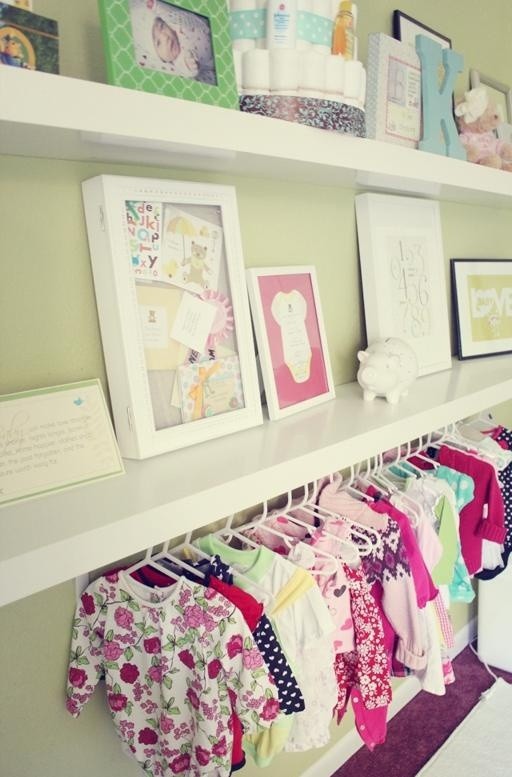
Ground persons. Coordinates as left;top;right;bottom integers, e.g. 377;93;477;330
133;8;214;77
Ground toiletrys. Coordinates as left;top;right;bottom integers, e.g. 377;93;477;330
266;0;293;49
332;0;354;61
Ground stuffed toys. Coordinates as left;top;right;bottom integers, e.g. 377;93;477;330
454;88;512;172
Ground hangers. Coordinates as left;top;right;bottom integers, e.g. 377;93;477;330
125;419;507;602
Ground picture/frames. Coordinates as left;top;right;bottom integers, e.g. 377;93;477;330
245;265;337;420
96;0;240;110
389;9;511;130
451;256;511;362
83;175;264;461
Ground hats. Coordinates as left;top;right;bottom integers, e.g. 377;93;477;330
132;9;164;64
